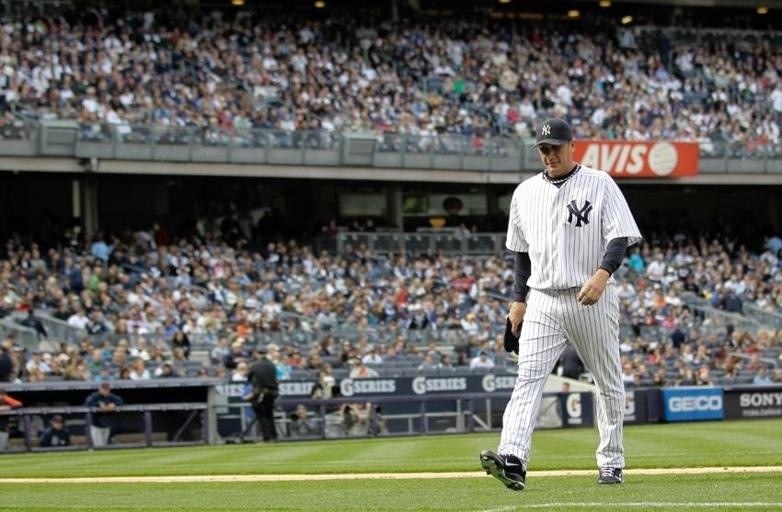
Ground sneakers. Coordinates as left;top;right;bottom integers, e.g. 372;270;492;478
597;467;623;485
479;450;528;491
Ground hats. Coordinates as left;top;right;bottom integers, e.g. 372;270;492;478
531;117;573;149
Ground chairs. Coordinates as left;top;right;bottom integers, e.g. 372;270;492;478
0;258;782;389
